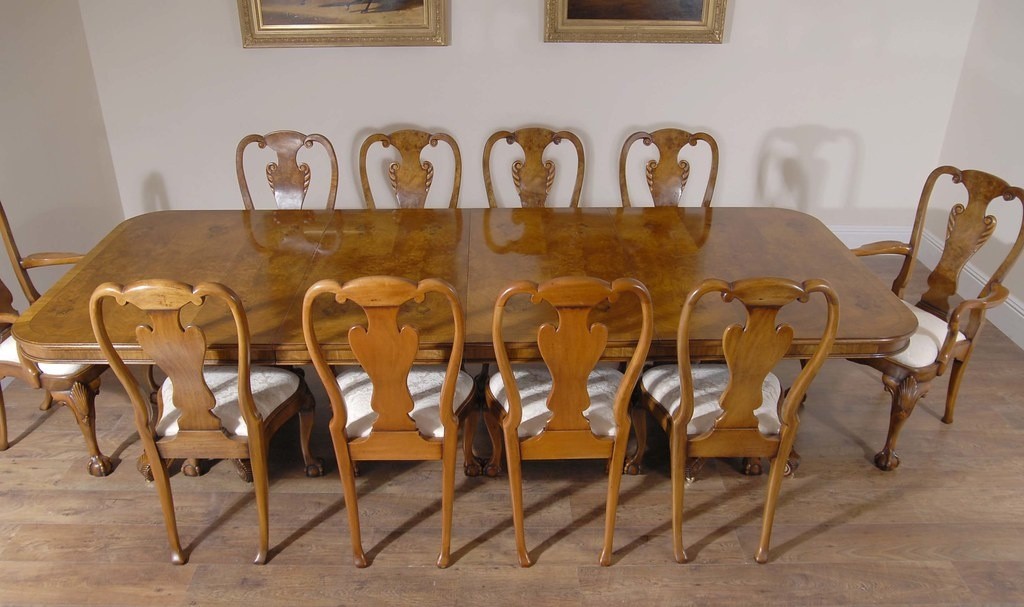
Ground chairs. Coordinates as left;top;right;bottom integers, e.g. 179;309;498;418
482;128;585;210
302;276;485;567
480;276;654;568
784;166;1024;470
619;128;719;208
236;130;338;209
625;276;839;562
0;200;114;477
358;129;462;210
89;279;329;566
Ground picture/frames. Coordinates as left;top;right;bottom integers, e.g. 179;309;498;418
543;0;727;44
236;0;448;48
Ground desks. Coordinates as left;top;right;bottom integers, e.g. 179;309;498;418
11;205;918;482
